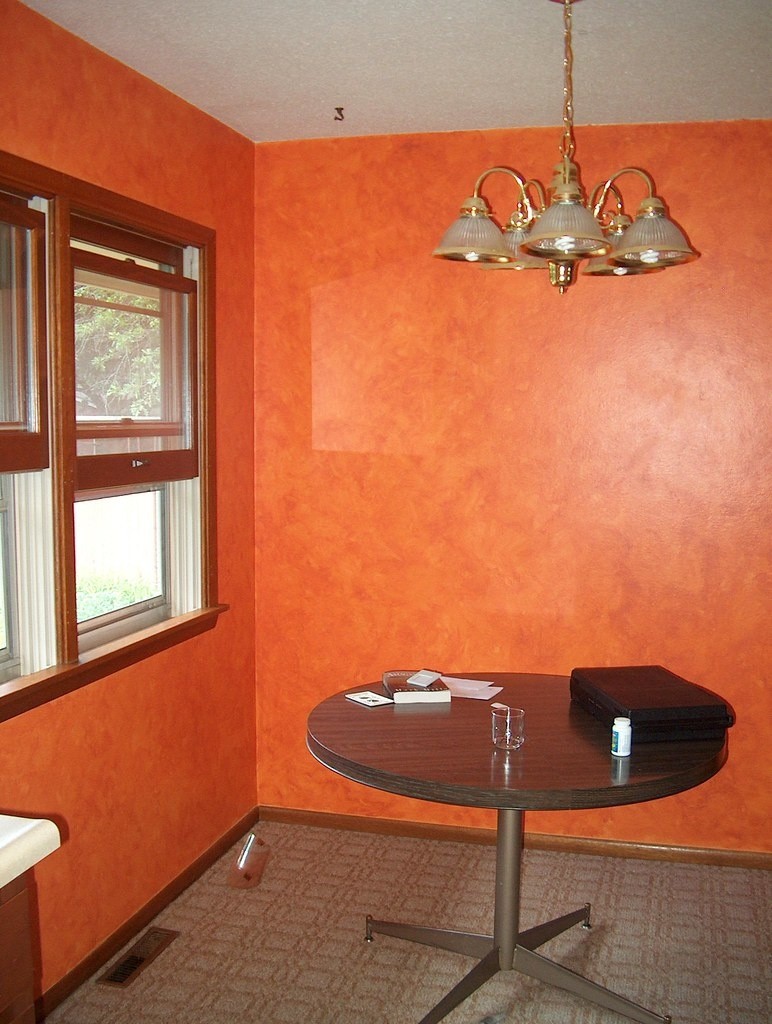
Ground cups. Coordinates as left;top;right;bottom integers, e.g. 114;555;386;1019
492;707;526;750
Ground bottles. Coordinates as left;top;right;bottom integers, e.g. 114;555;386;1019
229;830;270;888
611;717;632;757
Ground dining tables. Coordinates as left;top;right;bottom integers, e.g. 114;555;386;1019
305;672;729;1024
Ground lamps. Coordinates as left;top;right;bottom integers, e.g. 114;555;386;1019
431;0;701;295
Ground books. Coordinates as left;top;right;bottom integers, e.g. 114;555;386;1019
382;670;452;703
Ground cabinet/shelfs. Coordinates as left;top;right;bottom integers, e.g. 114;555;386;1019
1;813;60;1024
